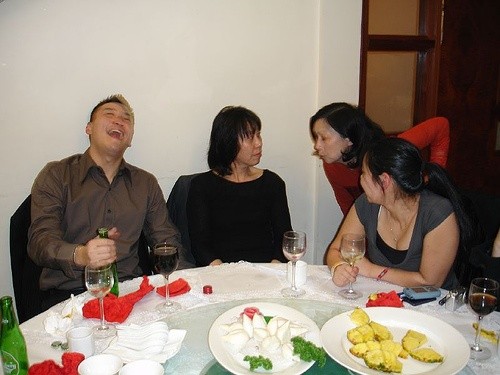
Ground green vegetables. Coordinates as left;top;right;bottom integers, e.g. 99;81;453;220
291;336;326;368
243;355;273;371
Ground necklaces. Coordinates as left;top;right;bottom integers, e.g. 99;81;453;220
389;222;398;241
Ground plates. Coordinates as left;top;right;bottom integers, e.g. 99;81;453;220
208;302;321;375
320;307;469;375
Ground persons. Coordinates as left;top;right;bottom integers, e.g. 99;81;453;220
310;101;451;216
327;137;470;288
186;105;295;268
26;95;181;309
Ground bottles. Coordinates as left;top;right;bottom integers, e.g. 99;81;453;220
97;227;119;298
0;295;29;375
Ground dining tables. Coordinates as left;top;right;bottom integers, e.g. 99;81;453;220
0;262;500;375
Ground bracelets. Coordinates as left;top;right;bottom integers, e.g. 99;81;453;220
331;262;349;278
377;267;391;281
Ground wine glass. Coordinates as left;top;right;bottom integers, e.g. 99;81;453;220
153;242;182;314
85;265;116;336
281;231;306;298
467;278;499;360
338;233;366;300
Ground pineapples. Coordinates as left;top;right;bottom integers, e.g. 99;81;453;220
347;308;444;373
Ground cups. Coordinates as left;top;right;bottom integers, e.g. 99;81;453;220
66;327;95;358
119;360;165;375
78;354;124;375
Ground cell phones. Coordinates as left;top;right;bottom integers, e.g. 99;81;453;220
398;286;441;299
